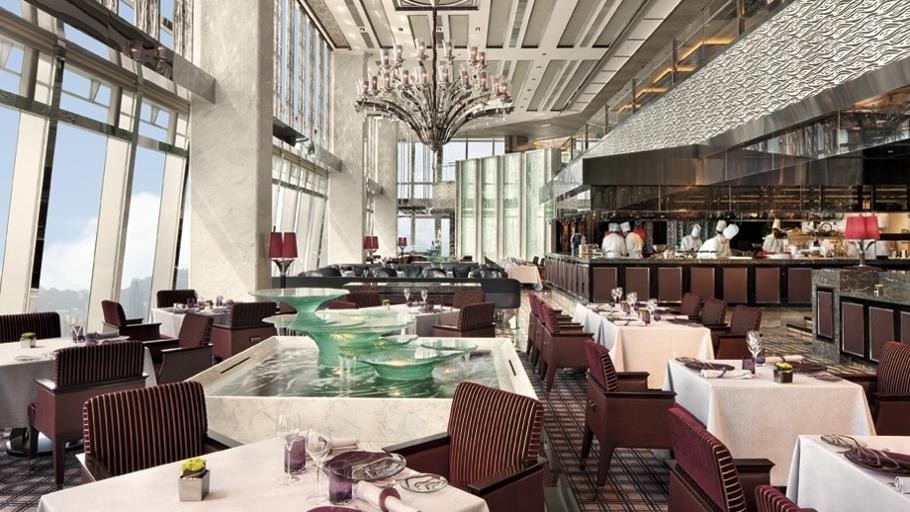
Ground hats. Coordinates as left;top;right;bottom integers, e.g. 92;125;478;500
608;223;620;232
716;219;727;232
620;222;631;233
722;224;739;240
691;225;702;238
772;219;781;229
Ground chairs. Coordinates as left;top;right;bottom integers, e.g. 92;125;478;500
708;305;762;360
383;382;548;512
669;409;787;512
143;313;213;388
537;258;546;282
838;342;910;437
27;341;145;483
533;299;581;364
526;293;563;354
671;293;702;319
330;302;356;310
213;302;277;363
533;256;537;265
491;264;505;278
0;312;60;345
538;304;595;391
347;291;381;307
689;297;728;328
432;301;495;338
452;289;485;309
157;290;197;309
101;301;160;342
77;382;207;484
755;484;812;512
485;258;499;278
580;341;679;496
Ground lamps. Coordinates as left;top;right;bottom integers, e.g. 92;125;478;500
364;237;379;263
398;238;408;257
270;232;297;290
355;8;514;152
844;216;881;270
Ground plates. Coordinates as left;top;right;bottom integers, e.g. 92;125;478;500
401;472;445;492
324;451;406;481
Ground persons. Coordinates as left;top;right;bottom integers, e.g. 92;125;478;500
715;220;725;235
681;223;703;255
696;224;739;257
601;223;626;257
762;217;781;254
621;221;644;257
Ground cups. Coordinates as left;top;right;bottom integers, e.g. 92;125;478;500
284;434;304;473
329;458;352;504
21;338;32;359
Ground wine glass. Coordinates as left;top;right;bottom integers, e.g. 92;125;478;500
72;315;82;345
67;315;75;338
421;288;428;304
626;292;637;317
309;429;329;502
647;298;658;320
404;287;411;304
611;288;623;309
747;330;765;376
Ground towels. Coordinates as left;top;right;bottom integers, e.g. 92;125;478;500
418;304;435;311
853;449;910;472
407;300;434;307
766;355;803;364
354;481;421;512
893;475;910;495
615;320;645;325
432;305;454;312
599;311;627;317
331;436;359;451
661;315;688;320
701;369;752;380
98;331;120;340
